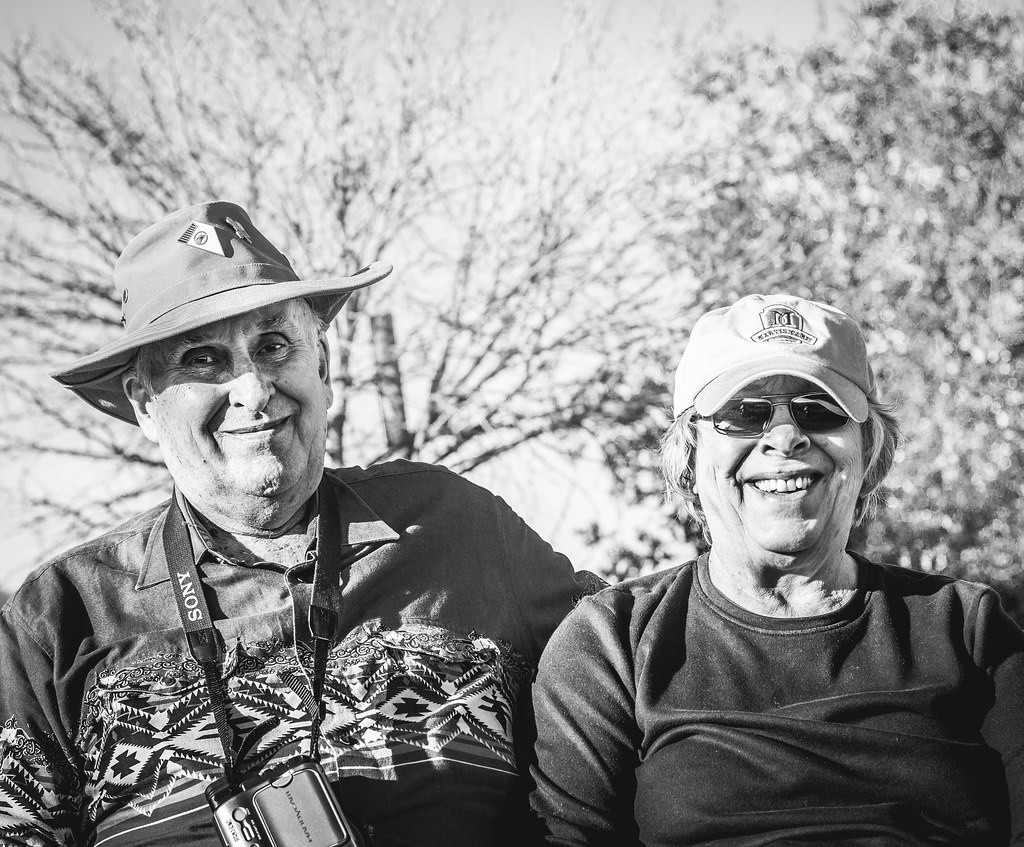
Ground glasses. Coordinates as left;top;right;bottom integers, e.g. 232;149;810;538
690;392;848;436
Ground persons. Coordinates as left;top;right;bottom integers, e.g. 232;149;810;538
0;201;607;846
531;295;1024;847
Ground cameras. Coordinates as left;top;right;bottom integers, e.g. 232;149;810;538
205;756;371;846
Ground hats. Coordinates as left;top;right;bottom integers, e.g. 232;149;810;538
50;202;394;427
668;294;875;425
